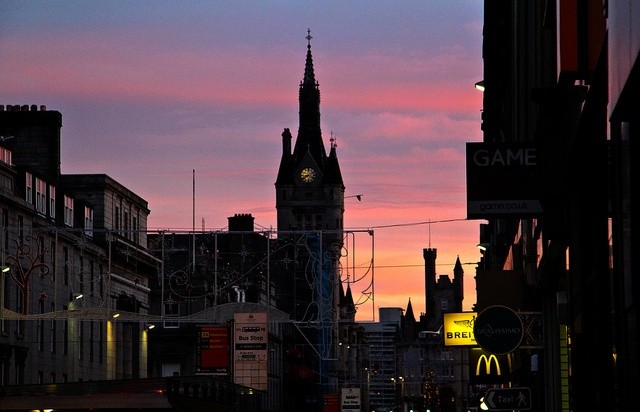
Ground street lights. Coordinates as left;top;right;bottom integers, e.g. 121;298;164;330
399;377;404;412
391;378;397;412
365;368;377;412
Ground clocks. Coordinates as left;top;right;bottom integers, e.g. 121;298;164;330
301;167;317;183
